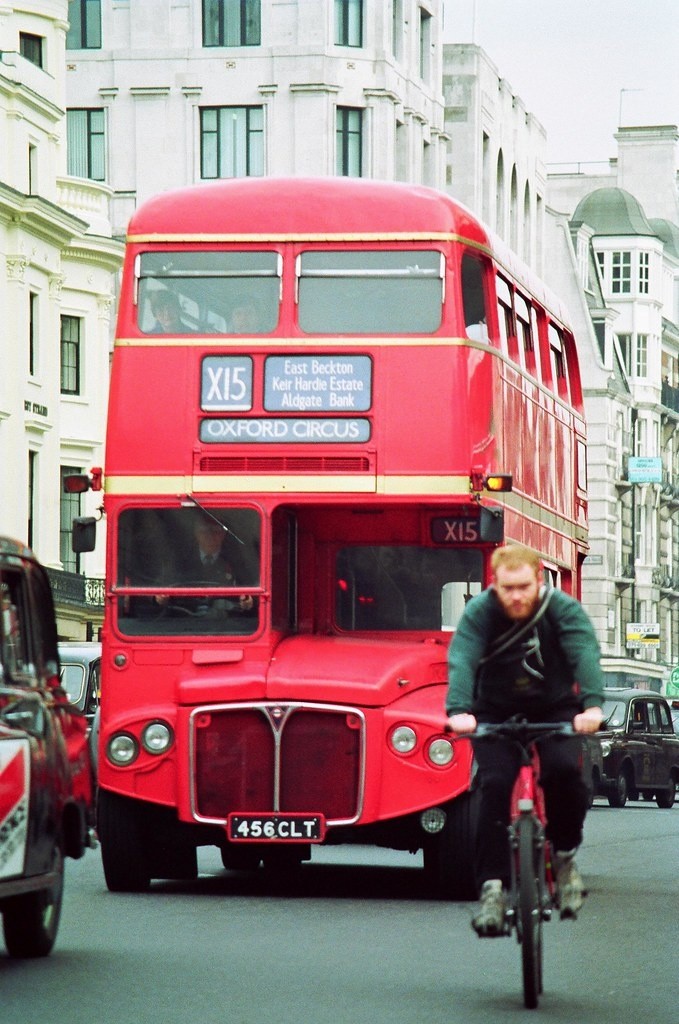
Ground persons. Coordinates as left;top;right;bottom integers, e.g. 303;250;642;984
442;541;606;935
147;516;256;618
143;289;191;335
229;301;264;334
144;513;258;619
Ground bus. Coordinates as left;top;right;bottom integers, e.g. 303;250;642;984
61;174;592;895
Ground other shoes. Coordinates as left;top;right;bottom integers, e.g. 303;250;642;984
557;866;585;918
470;883;505;935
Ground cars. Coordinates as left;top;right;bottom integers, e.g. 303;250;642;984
0;534;103;962
579;682;679;810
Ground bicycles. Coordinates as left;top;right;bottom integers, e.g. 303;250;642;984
442;717;618;1012
